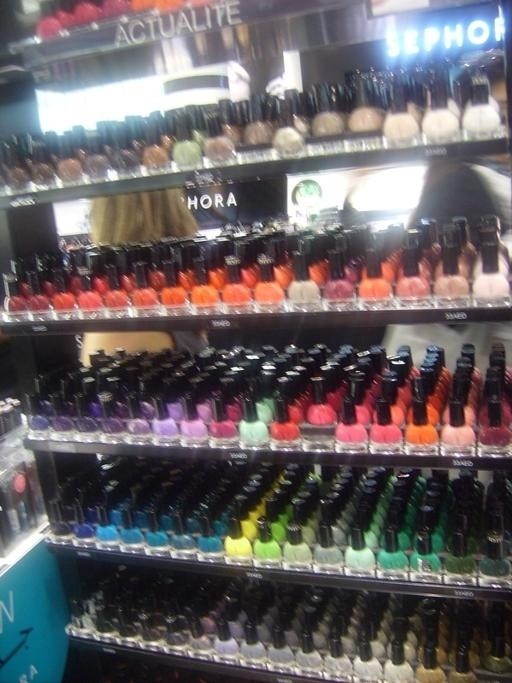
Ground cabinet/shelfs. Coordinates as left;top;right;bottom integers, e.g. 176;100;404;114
0;0;511;680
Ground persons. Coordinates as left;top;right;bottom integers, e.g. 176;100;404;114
80;188;199;368
409;48;512;238
